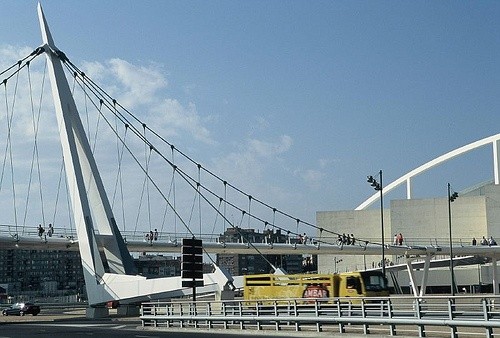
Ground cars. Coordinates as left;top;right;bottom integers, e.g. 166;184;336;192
2;302;40;316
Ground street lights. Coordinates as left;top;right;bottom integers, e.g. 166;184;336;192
334;256;343;273
448;183;459;318
367;170;385;275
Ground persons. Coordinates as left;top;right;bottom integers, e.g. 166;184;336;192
338;233;355;245
146;229;158;240
393;234;398;245
472;238;476;245
47;224;54;237
37;224;43;237
298;232;308;244
481;236;497;245
398;233;402;245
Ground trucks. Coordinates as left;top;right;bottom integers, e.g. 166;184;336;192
244;269;393;316
